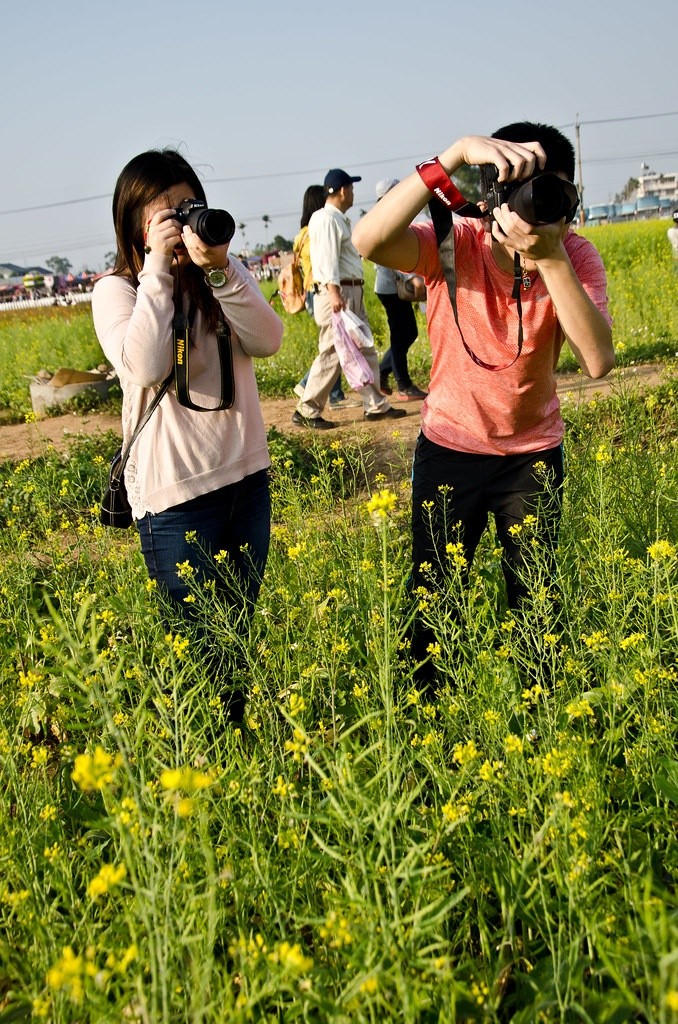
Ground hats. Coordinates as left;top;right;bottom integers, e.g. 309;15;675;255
376;178;400;199
324;169;362;195
672;208;678;224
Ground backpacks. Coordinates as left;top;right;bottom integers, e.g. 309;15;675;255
277;231;308;315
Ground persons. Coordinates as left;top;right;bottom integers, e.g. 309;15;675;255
373;177;426;398
664;209;678;250
293;183;360;408
352;122;614;692
90;148;286;723
292;169;407;430
237;254;249;268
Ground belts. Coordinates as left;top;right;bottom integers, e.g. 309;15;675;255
340;278;365;286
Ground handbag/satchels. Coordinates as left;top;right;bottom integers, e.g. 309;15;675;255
396;276;428;301
332;307;374;392
99;447;134;529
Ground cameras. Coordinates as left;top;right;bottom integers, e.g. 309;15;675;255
157;197;236;252
481;158;581;241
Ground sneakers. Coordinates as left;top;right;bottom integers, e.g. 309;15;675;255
396;386;430;400
380;384;393;396
293;385;306;397
329;396;363;409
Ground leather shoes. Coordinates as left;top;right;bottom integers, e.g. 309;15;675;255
364;405;406;421
292;411;334;429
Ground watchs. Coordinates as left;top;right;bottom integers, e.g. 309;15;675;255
204;265;230;289
